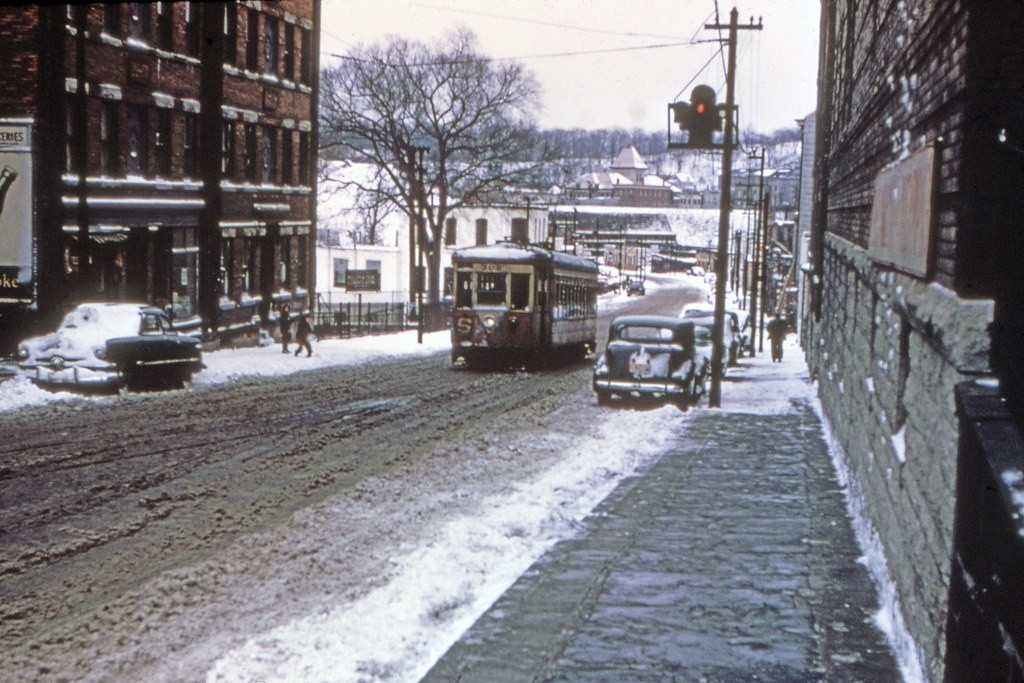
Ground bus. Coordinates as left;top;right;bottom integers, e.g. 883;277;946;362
449;241;599;366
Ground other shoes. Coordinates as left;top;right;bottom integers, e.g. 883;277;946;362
295;350;298;356
307;350;312;357
282;348;290;354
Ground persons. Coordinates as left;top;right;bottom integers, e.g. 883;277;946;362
767;313;787;363
280;304;295;353
295;315;312;357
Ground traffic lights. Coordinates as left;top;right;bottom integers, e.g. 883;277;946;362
688;86;716;144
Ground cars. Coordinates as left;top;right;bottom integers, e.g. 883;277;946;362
589;314;710;412
626;282;645;296
658;325;730;378
685;309;751;367
15;302;202;394
